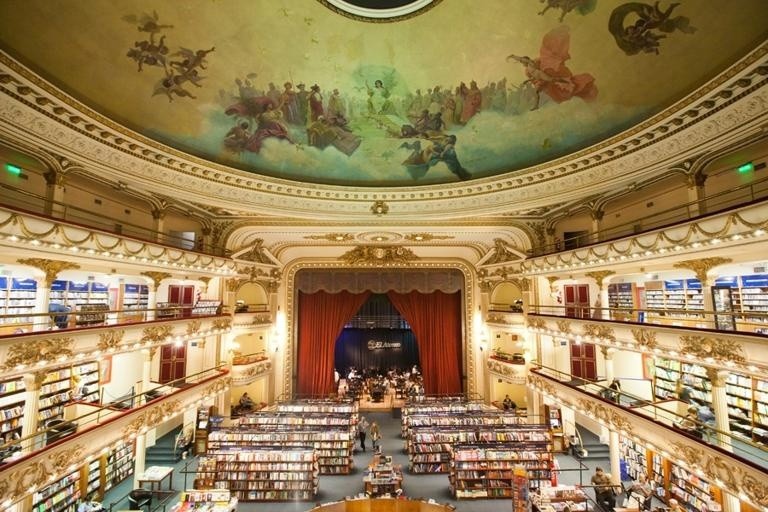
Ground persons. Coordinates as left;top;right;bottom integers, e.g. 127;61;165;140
503;394;514;410
240;392;252;405
126;21;216;102
569;435;581;446
622;23;667;58
636;0;682;34
610;378;622;395
358;416;369;451
592;466;617;504
369;422;382;450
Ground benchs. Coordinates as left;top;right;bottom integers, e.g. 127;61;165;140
752;427;768;444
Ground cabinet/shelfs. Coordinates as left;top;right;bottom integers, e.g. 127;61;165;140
618;435;722;511
0;358;137;511
606;271;768;322
0;275;149;337
190;399;563;500
653;354;767;431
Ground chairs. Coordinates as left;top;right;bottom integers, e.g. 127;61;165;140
128;488;153;512
144;390;163;404
156;301;174;320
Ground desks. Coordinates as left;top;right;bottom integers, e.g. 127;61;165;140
138;467;175;500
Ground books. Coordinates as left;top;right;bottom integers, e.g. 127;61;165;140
654;358;768;439
0;290;150;512
449;446;595;511
611;289;768;324
402;397;526;453
208;429;355;473
180;453;320;512
617;435;723;510
241;400;358;436
410;429;551;473
